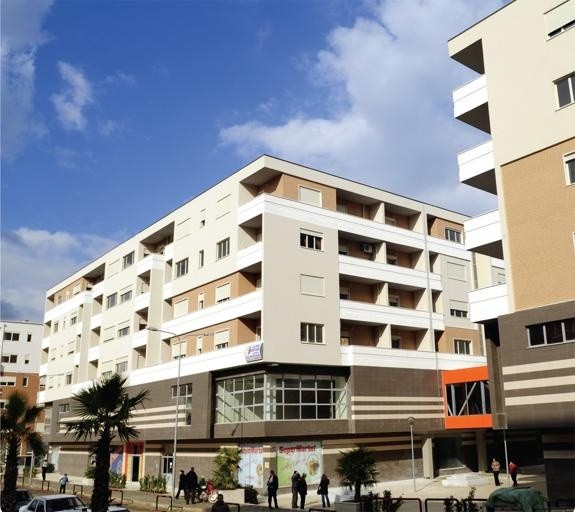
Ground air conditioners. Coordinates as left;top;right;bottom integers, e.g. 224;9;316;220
361;244;372;253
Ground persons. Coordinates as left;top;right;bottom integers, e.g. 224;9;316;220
508;461;524;488
184;467;198;504
491;458;501;486
319;474;330;507
41;460;48;481
58;474;68;493
296;473;307;510
266;470;278;508
212;494;230;512
292;471;300;508
175;470;187;499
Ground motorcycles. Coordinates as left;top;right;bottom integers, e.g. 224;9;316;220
197;486;218;504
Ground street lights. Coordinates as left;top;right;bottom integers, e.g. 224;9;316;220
146;326;214;507
408;418;416;492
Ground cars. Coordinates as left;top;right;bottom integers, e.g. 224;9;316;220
15;488;129;512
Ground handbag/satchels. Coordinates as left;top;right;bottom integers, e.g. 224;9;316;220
317;488;321;494
